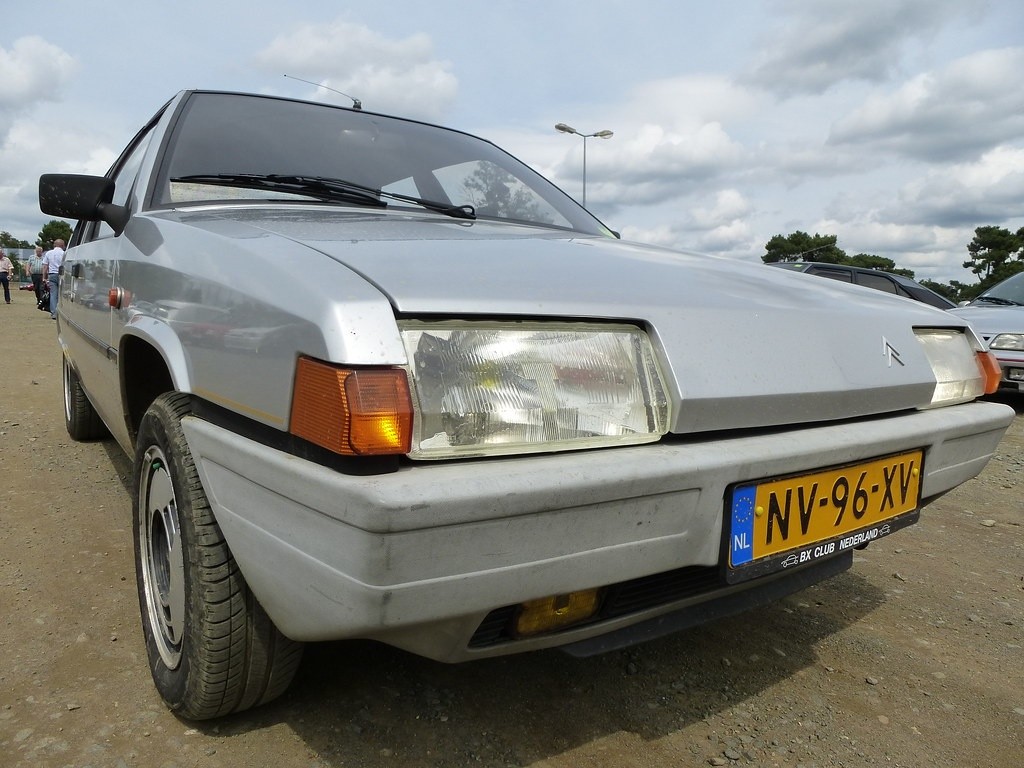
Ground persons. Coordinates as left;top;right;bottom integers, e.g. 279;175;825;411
26;247;47;305
42;239;65;318
0;247;15;303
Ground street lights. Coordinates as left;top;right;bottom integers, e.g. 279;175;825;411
555;122;615;211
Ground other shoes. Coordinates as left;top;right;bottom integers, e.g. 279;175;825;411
6;300;10;304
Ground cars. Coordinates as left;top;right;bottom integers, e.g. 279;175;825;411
20;281;50;291
38;87;1019;718
113;297;307;357
944;271;1024;392
63;289;111;312
765;261;958;310
